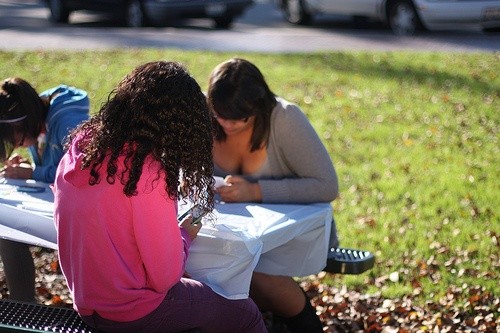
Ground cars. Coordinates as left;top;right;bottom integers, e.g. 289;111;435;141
280;1;499;36
42;1;255;31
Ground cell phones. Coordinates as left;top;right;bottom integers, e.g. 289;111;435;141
212;176;226;188
177;204;209;224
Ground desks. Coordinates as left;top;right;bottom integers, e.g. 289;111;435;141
0;169;332;301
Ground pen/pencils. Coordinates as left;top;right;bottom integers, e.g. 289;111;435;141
0;161;18;173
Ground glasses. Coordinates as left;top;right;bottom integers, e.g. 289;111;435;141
213;106;252;123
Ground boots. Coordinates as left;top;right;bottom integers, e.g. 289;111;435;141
286;299;324;333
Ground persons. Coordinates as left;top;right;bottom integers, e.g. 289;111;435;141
0;79;93;305
52;60;268;333
200;57;338;333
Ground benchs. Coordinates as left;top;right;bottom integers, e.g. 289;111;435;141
0;298;97;333
323;248;375;274
